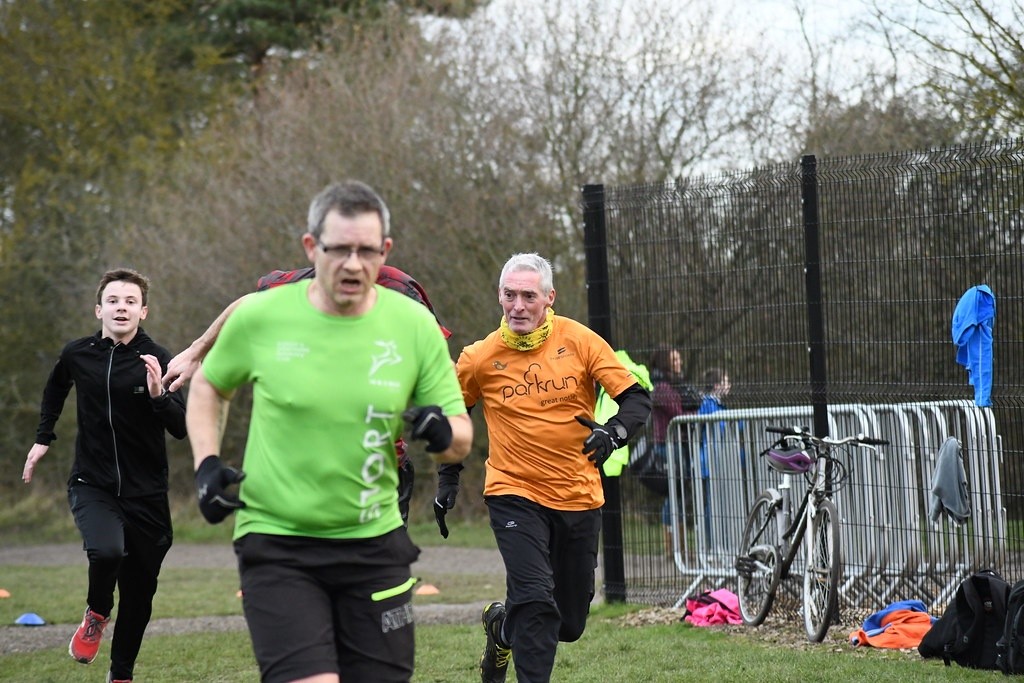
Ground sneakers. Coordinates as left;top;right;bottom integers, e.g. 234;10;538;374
105;671;132;683
479;601;512;683
68;606;111;664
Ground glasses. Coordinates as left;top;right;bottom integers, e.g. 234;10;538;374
312;238;386;259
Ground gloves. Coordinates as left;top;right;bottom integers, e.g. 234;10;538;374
194;455;246;525
401;404;454;454
574;416;627;468
432;463;465;539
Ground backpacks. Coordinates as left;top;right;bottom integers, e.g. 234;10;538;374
918;566;1024;675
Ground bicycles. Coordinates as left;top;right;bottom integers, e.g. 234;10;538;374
734;425;892;646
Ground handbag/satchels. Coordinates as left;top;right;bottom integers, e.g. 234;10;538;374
642;453;688;497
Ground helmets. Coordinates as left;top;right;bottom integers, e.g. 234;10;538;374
763;447;816;475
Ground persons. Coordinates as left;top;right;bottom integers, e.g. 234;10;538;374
435;252;654;683
182;177;477;683
627;341;703;560
23;267;185;683
158;258;450;529
691;369;751;558
